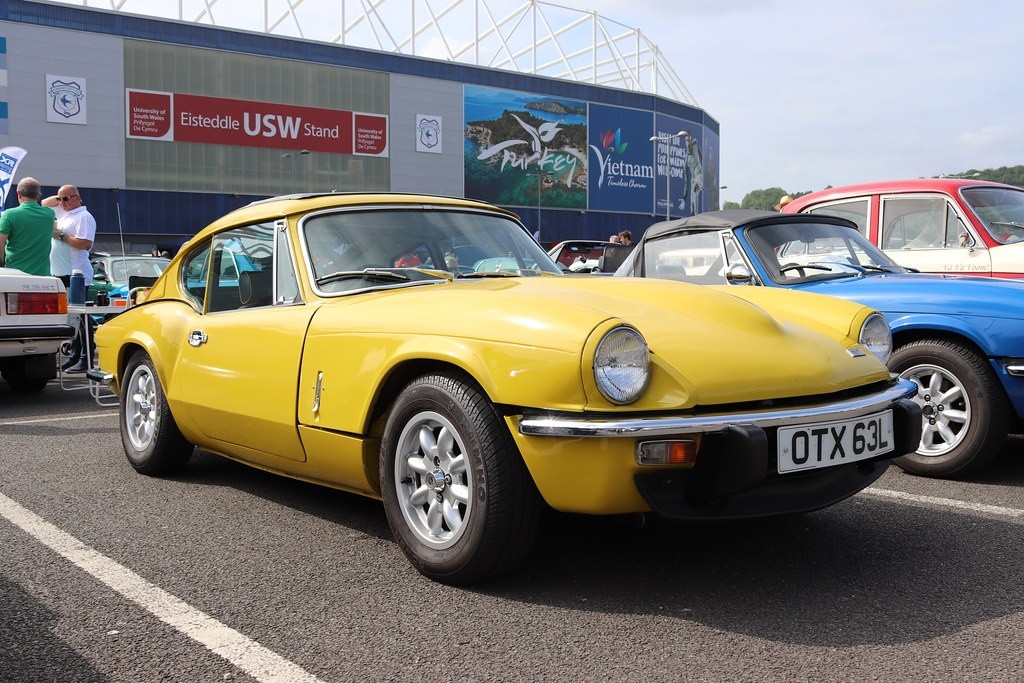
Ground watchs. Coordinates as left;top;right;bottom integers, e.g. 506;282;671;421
58;232;63;242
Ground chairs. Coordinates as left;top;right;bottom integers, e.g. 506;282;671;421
236;267;274;309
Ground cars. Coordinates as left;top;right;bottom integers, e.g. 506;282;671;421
181;237;275;291
766;176;1023;283
445;237;629;278
0;267;75;395
84;248;180;301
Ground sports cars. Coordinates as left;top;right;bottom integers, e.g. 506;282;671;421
608;207;1024;476
83;187;924;587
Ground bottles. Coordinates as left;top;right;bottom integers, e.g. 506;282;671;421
96;290;109;306
69;269;85;305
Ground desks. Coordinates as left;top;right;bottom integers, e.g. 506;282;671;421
55;306;134;408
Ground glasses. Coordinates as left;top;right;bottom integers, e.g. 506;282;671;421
56;195;78;201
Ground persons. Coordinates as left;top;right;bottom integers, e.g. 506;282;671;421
0;176;55;276
150;248;158;258
41;183;97;295
608;234;619;247
442;252;460;279
678;124;702;218
618;229;637;248
397;251;420;270
161;249;173;266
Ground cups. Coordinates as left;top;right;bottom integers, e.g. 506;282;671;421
85;301;94;306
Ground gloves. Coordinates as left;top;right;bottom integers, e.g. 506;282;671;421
678;198;686;210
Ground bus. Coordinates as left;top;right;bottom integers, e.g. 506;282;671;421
657;246;723;277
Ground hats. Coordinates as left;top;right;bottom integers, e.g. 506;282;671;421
774;196;793;210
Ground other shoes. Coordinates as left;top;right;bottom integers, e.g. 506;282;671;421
65;362;87;372
56;359;75;371
